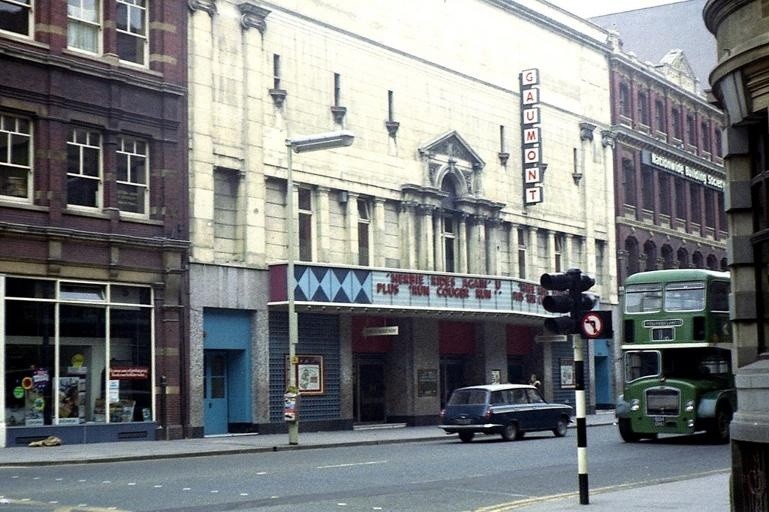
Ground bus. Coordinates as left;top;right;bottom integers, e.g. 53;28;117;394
611;268;738;445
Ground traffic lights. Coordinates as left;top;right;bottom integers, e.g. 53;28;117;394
579;272;598;312
538;267;584;338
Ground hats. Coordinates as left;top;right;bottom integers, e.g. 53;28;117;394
22;377;32;389
33;398;45;411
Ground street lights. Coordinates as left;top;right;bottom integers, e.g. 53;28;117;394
285;130;355;446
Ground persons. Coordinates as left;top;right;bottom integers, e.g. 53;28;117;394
527;374;542;388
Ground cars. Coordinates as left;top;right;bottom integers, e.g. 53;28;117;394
436;383;574;442
